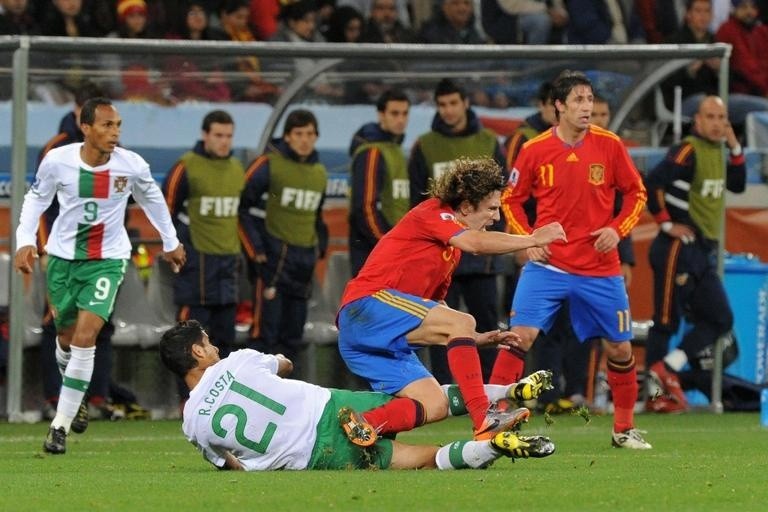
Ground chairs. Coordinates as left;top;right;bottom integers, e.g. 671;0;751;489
2;240;352;420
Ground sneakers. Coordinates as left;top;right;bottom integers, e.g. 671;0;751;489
647;395;680;412
45;425;65;452
107;404;149;420
473;407;529;440
505;370;554;400
72;400;89;433
649;361;685;405
491;431;556;464
611;429;651;449
339;406;377;446
